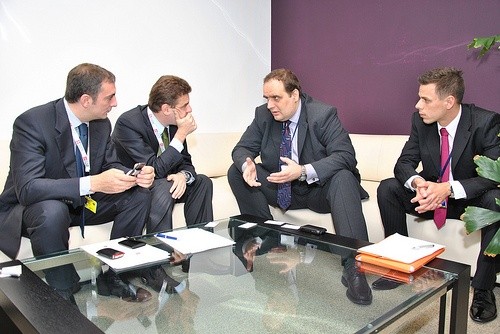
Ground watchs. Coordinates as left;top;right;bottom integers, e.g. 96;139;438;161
298;164;306;182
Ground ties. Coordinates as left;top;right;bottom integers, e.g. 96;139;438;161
156;127;170;157
76;123;89;238
434;128;450;229
276;119;292;211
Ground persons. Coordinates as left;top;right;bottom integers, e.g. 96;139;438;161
108;74;250;294
227;69;374;306
372;65;500;323
0;62;153;315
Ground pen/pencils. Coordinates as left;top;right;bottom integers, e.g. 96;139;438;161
413;245;434;249
157;234;177;240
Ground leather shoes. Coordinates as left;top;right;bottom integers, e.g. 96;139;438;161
340;260;373;305
55;288;80;313
95;273;153;303
128;265;184;294
469;289;497;323
182;255;231;275
372;277;401;290
254;234;281;253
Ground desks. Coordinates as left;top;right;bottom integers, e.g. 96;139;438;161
0;213;472;334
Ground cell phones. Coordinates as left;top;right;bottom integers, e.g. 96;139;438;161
96;248;124;260
300;224;327;234
118;239;146;248
126;162;145;177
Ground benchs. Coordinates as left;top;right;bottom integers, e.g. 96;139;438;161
0;134;500;285
